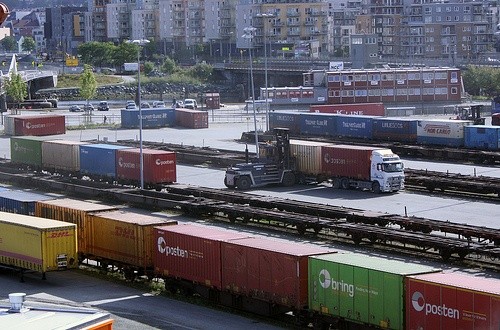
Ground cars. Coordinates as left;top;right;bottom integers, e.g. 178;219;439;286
84;103;95;111
154;99;166;109
184;98;195;109
98;100;109;111
126;100;136;110
69;105;82;112
141;100;151;108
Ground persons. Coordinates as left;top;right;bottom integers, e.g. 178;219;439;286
104;115;107;123
173;98;176;108
452;105;458;115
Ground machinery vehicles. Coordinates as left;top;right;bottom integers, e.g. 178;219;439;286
449;102;485;124
224;126;297;190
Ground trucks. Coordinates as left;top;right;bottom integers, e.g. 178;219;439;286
124;62;145;74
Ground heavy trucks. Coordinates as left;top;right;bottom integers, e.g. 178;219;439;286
255;140;405;194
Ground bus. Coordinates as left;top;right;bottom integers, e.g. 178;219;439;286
25;99;58;107
8;103;53;110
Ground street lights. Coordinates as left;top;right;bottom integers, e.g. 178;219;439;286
241;26;260;161
252;12;276;133
124;39;151;191
